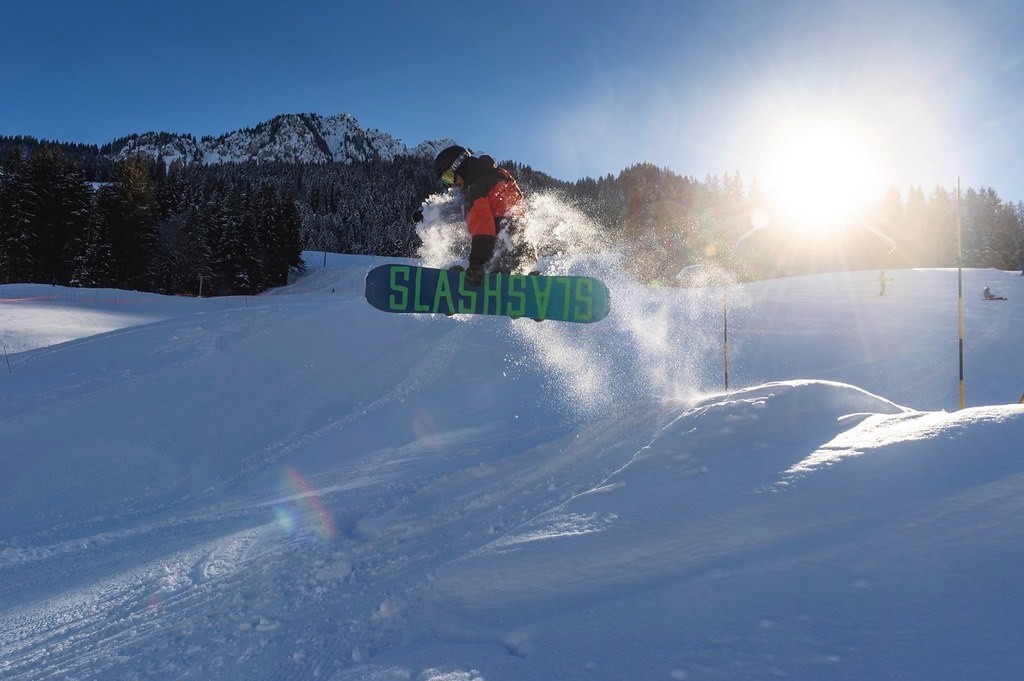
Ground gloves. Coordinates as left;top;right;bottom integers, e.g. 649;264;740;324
465;258;486;285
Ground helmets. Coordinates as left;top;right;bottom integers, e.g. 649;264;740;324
434;146;469;181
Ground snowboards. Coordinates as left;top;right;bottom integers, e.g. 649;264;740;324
364;263;611;323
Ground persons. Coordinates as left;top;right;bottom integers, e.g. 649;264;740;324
412;146;546;322
983;287;995;300
878;271;893;296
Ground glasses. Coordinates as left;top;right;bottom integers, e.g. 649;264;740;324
437;168;457;192
